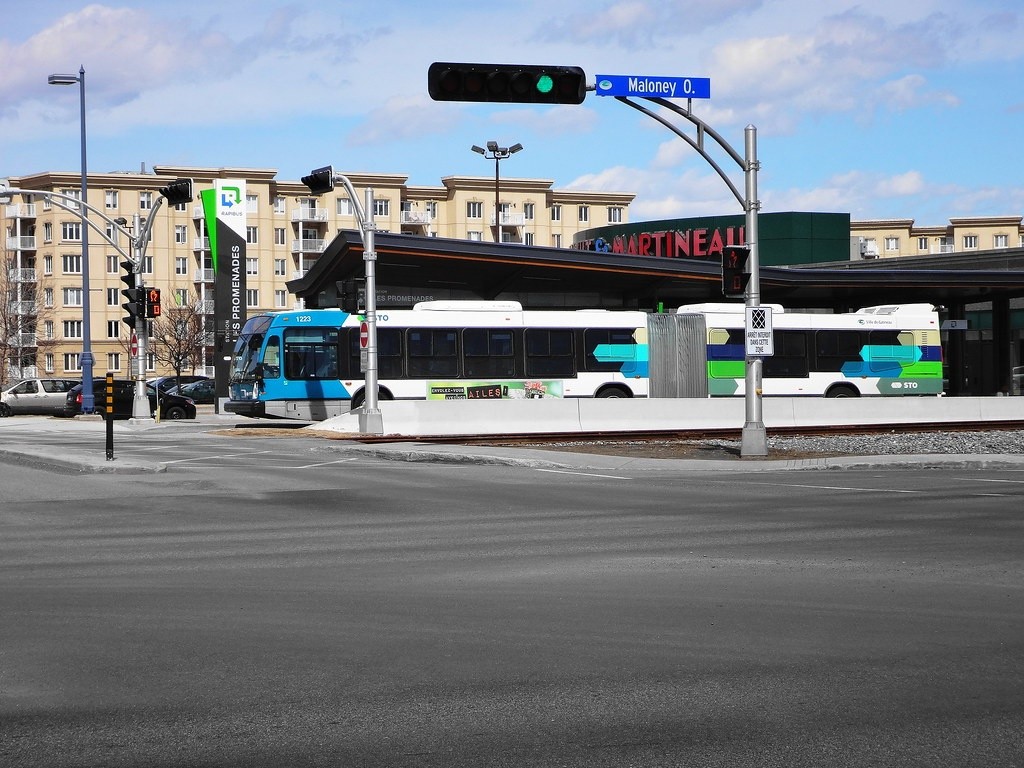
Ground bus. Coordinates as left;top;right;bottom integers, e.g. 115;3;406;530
216;300;947;421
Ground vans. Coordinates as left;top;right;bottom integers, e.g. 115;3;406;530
0;379;83;418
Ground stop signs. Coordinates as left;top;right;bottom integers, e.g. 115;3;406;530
359;321;370;349
131;335;138;356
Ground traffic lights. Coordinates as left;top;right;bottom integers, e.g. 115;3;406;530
144;286;161;319
119;258;144;329
335;279;356;313
300;165;335;196
428;61;588;106
721;246;752;299
159;178;194;207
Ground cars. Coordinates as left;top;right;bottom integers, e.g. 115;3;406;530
64;378;197;420
146;375;215;404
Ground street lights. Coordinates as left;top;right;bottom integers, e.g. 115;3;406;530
470;141;524;243
48;64;96;415
